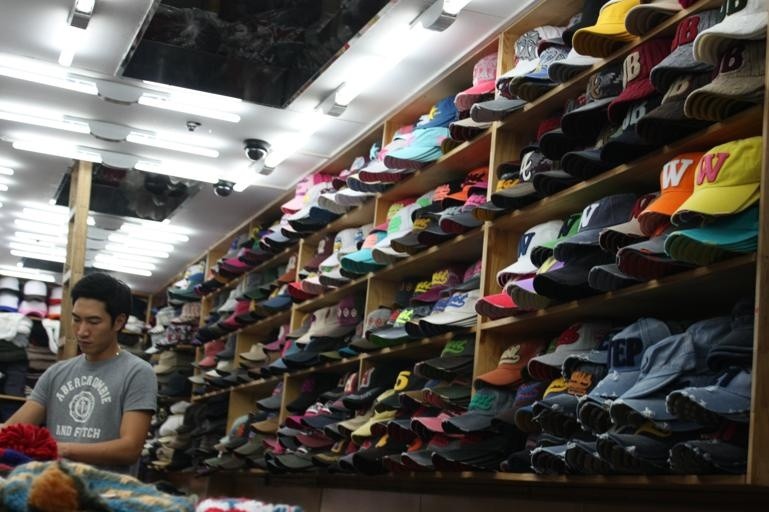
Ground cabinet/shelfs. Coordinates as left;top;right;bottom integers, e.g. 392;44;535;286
208;181;302;472
470;1;768;488
361;58;472;484
302;137;361;483
117;289;152;358
0;274;64;397
148;252;207;478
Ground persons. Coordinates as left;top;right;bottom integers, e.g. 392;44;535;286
0;272;155;480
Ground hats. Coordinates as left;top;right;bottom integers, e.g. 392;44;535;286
233;248;300;335
195;227;253;301
270;298;361;379
349;257;480;359
223;314;291;391
204;382;282;475
170;396;226;474
266;368;360;474
338;165;488;286
442;322;751;480
475;219;761;329
143;259;204;350
239;186;303;279
337;335;476;480
0;278;67;320
347;50;497;208
478;0;769;220
289;137;384;245
188;281;236;348
187;334;236;398
137;400;189;478
287;217;376;310
151;345;195;399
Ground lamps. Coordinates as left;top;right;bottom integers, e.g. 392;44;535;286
232;2;468;194
0;106;219;162
1;54;241;126
12;131;219;192
314;0;469;116
56;1;98;67
9;201;190;278
235;132;317;195
